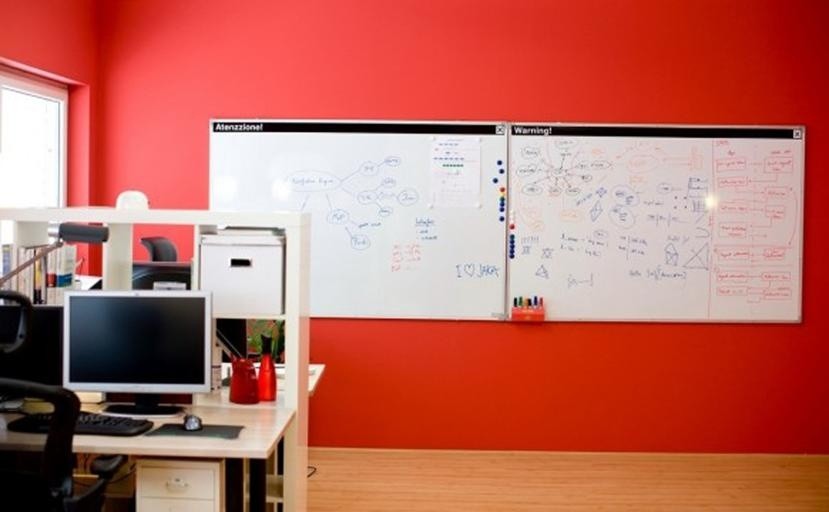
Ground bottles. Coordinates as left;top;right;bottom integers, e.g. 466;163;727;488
227;355;259;404
258;331;277;402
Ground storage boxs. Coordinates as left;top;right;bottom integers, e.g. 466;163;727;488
197;230;284;318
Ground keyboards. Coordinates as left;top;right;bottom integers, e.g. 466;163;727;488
9;410;152;436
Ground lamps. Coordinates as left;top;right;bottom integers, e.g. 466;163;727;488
0;220;112;293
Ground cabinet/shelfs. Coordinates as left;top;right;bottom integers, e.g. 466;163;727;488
0;207;311;509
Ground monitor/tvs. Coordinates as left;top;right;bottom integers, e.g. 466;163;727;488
133;263;248;363
0;305;62;395
63;292;216;419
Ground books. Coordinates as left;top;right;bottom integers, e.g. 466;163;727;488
2;240;79;306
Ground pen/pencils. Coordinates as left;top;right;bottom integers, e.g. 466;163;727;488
513;296;546;306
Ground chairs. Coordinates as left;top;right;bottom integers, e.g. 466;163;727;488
1;375;129;512
132;236;191;290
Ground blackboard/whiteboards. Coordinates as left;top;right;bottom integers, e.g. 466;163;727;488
207;117;803;323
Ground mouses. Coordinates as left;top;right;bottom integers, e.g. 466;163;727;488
184;416;202;430
